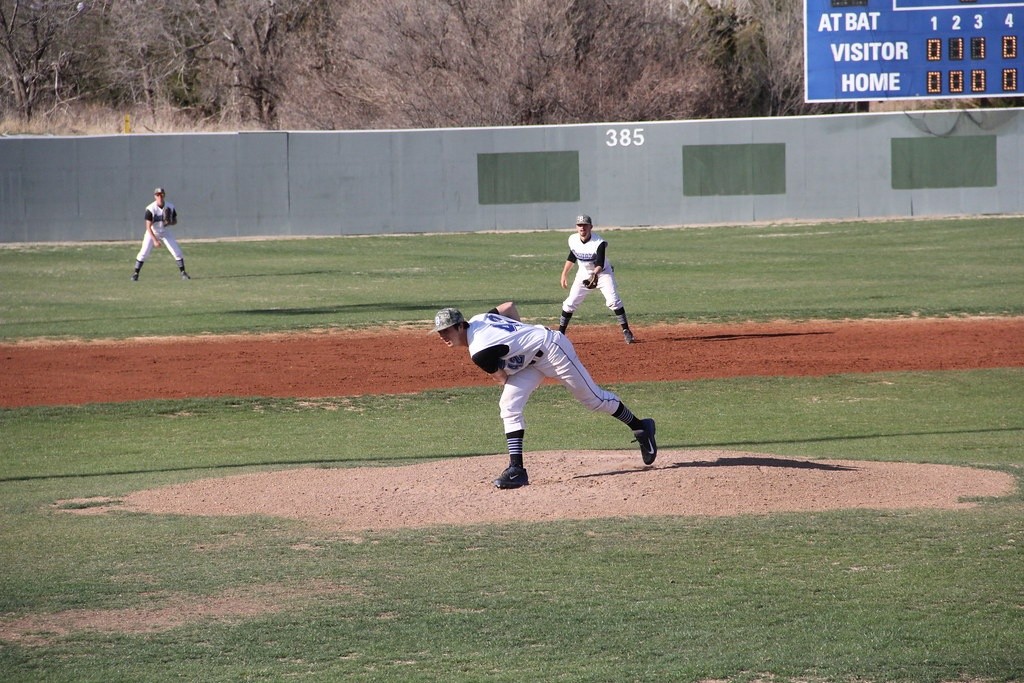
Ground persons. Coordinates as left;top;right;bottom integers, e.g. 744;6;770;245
558;214;634;345
130;188;190;281
432;301;657;488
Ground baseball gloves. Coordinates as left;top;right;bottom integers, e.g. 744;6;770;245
162;207;177;226
583;273;598;289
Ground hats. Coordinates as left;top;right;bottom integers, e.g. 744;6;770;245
430;307;464;333
154;188;164;194
576;214;592;225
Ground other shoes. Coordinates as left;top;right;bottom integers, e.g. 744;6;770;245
181;271;190;279
131;272;139;281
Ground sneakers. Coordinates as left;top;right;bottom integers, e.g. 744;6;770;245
630;418;657;465
623;329;635;344
494;466;529;488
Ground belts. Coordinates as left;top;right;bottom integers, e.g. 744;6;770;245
529;350;544;364
